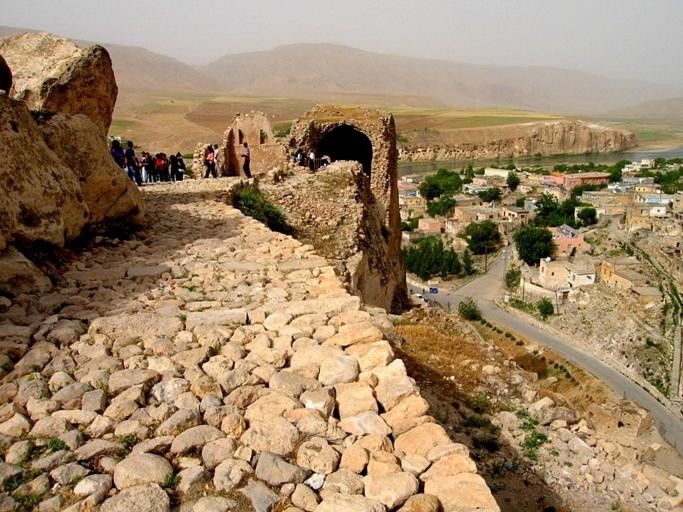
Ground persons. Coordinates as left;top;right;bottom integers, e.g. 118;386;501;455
110;140;125;169
294;146;316;173
134;151;186;182
125;141;145;186
321;154;330;167
214;145;222;178
240;142;252;178
204;146;217;178
